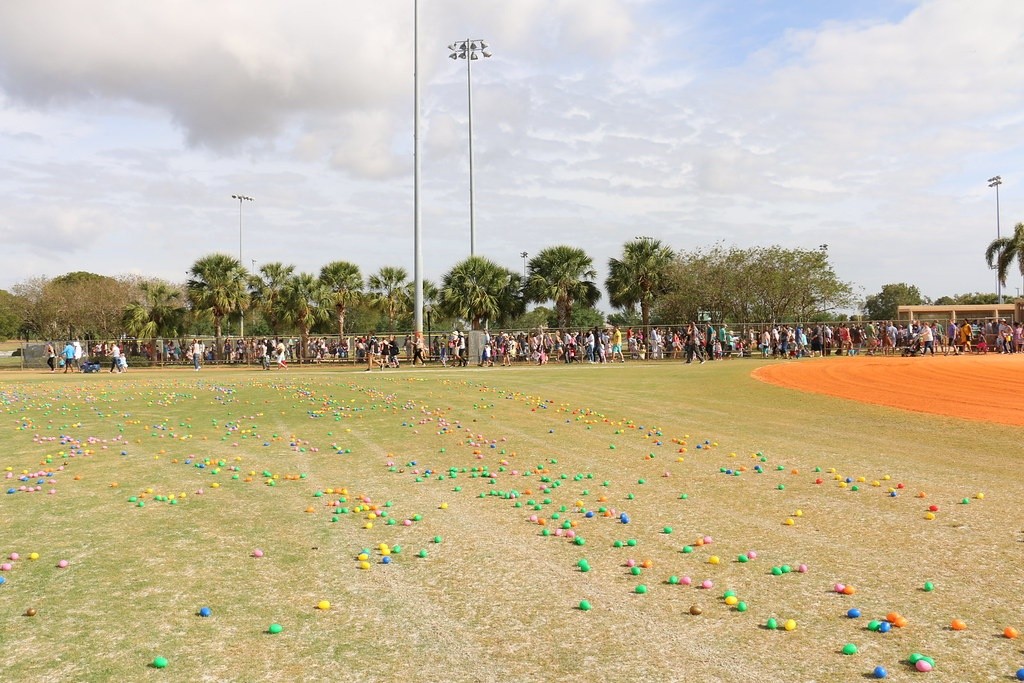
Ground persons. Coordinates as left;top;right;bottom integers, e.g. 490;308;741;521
46;319;1024;372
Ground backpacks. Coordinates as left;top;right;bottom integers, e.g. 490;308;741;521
834;328;840;340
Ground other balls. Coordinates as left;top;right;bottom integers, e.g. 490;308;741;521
962;498;969;504
0;376;939;683
977;493;984;499
1016;669;1024;680
1005;625;1018;639
952;619;965;630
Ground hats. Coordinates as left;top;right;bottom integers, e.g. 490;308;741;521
898;325;903;328
1001;319;1006;321
453;331;458;335
460;332;464;336
933;319;939;323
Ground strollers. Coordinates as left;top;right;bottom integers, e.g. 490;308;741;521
899;334;921;357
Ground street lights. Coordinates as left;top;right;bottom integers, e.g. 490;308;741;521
987;175;1003;304
231;193;255;339
819;243;829;320
635;236;654;242
520;251;528;286
447;38;491;256
425;304;433;360
991;265;998;303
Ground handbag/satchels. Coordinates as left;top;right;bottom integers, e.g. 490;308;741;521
416;339;422;349
391;345;400;355
672;342;677;347
277;347;283;355
532;350;541;361
453;342;459;355
966;335;972;340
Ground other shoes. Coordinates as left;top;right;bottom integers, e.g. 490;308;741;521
365;368;371;371
380;364;383;370
196;366;201;371
700;361;705;365
685;363;690;365
